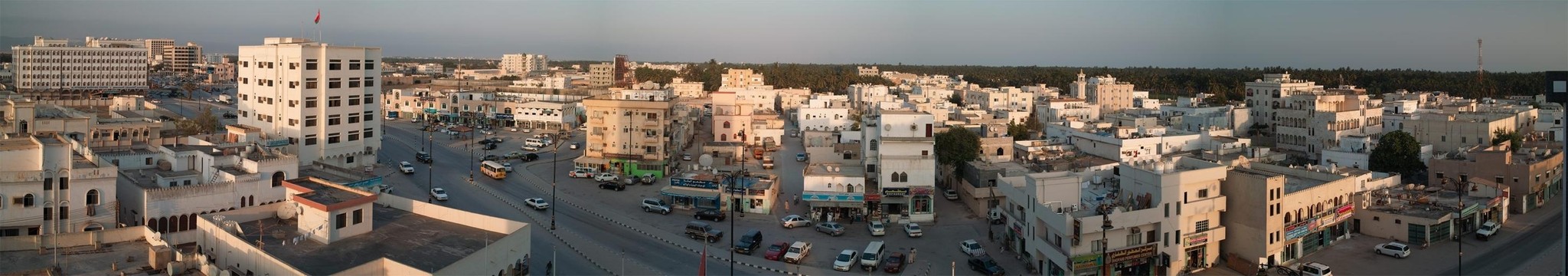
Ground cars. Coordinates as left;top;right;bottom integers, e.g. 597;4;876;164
524;197;549;211
477;123;494;135
533;121;587;150
884;251;906;273
833;249;859;272
479;149;539;172
687;95;694;98
207;98;213;102
868;221;886;236
704;112;712;116
958;239;986;258
431;188;448;201
411;116;460;135
780;214;812;229
520;145;540;152
903;223;923;238
944;189;958;200
694;209;726;222
146;75;203;104
814;221;845;237
796;152;805;162
968;255;1006;276
790;130;796;137
215;98;220;102
683;153;692;161
777;111;781;115
229;81;234;83
510;126;518;132
203;86;229;93
765;241;790;261
703;105;711;109
569;169;640;192
158;116;175;121
478;136;504;151
522;127;530;133
385;116;396;120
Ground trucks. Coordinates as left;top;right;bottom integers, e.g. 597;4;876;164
753;137;776;169
220;94;233;105
986;207;1004;225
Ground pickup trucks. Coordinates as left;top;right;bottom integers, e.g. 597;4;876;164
784;238;813;263
1476;219;1501;241
379;183;394;194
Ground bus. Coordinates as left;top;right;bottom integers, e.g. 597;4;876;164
480;160;506;179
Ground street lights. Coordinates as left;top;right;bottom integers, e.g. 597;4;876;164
494;90;498;136
628;109;633;175
481;101;489;160
428;86;431;126
551;131;558;230
468;110;477;181
737;127;746;217
1257;262;1303;276
1095;203;1114;275
1440;175;1479;276
421;95;425;151
427;118;435;203
383;89;386;135
118;223;126;228
712;163;750;275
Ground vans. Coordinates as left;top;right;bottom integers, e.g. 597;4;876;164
525;137;546;148
860;241;885;270
99;97;109;100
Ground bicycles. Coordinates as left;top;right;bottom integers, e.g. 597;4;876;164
1178;266;1193;276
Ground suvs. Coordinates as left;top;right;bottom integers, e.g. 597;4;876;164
734;229;763;256
1374;242;1411;259
223;113;237;119
683;219;725;243
398;161;415;174
641;172;656;185
416;151;433;164
1289;261;1333;276
641;197;673;215
